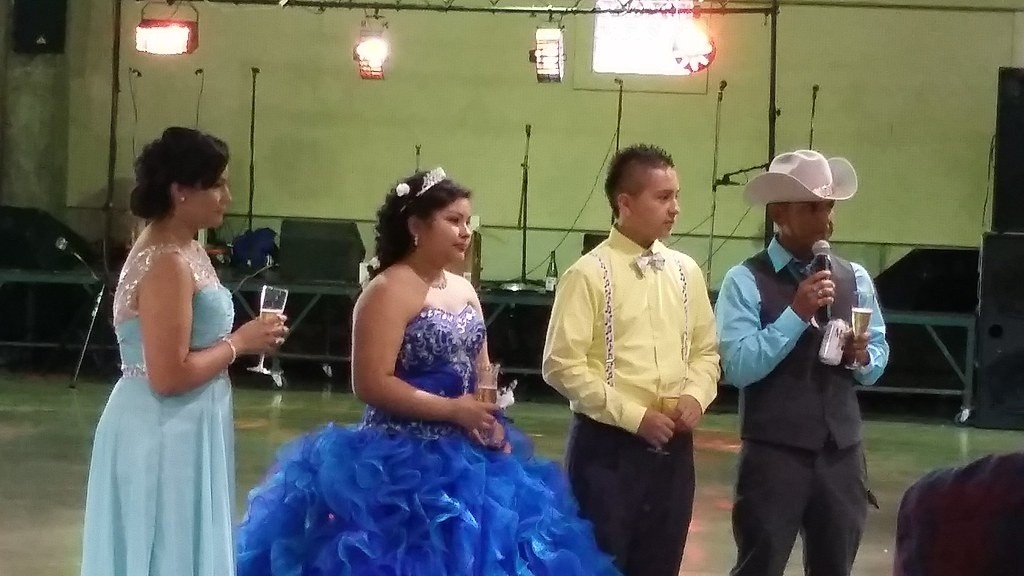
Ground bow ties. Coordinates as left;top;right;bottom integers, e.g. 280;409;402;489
633;252;665;278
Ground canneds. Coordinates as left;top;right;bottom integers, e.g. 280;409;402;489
819;318;849;366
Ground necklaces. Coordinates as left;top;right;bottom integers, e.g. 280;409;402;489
408;263;448;290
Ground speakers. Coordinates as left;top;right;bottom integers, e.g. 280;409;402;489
874;248;980;314
11;0;67;55
974;65;1024;432
0;206;97;271
277;219;367;283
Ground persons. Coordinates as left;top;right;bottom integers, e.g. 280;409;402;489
236;166;622;576
892;449;1024;576
83;128;289;576
713;151;890;576
541;143;720;576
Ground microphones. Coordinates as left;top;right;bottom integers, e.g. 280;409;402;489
812;84;819;92
716;178;742;186
812;240;833;326
719;81;727;91
614;78;623;84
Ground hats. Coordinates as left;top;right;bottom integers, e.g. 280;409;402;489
742;149;858;205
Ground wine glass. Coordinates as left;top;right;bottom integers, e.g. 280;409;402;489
246;284;288;374
844;305;873;371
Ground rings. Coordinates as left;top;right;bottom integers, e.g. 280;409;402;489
818;291;823;297
818;299;823;307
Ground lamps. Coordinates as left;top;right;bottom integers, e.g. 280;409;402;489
529;12;567;82
673;5;716;73
353;15;389;79
135;0;199;56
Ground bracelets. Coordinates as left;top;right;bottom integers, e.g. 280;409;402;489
222;338;236;363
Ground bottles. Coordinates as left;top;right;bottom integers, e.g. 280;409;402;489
545;251;558;293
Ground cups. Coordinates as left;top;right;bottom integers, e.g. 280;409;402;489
476;362;500;416
